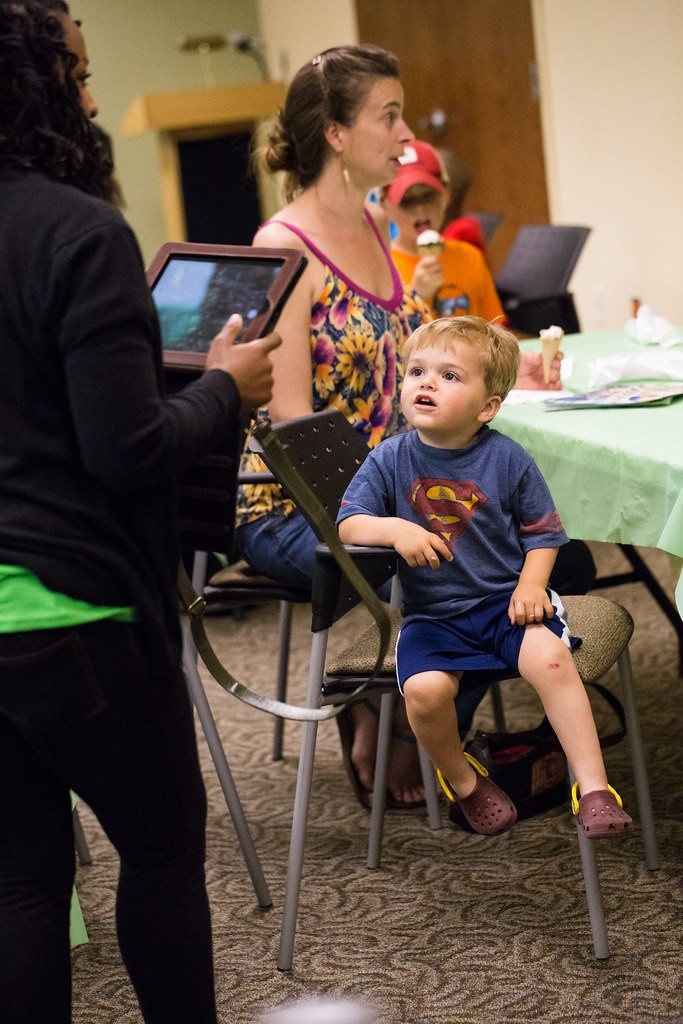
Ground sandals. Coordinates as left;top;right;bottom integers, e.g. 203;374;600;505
434;752;519;834
571;780;634;839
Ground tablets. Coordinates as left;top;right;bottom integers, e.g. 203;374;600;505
144;241;308;372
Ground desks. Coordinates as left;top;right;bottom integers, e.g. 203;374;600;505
479;323;682;732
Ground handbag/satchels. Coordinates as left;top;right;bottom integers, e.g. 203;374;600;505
448;725;571;834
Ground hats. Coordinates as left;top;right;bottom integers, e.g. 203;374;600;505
436;148;473;191
442;217;486;249
382;139;443;206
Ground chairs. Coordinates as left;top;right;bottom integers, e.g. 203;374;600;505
183;470;511;757
71;551;274;911
252;408;664;970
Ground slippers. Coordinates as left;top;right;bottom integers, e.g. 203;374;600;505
328;688;377;816
388;727;425;811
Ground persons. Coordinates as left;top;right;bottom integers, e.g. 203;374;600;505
232;45;565;814
335;315;633;839
372;137;509;329
0;0;281;1024
